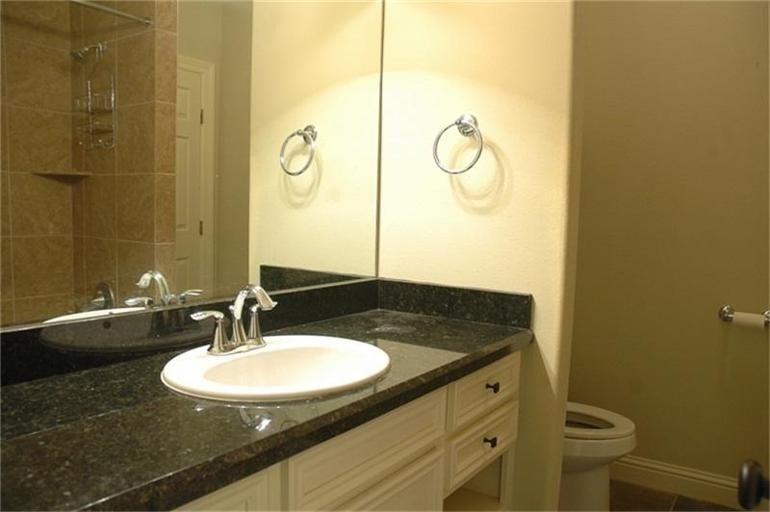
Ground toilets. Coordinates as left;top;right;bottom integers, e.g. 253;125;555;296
556;399;637;512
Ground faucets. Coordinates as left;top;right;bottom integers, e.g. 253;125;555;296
226;283;279;350
134;268;182;306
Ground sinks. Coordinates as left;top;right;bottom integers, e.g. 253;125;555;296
44;306;223;349
158;333;390;405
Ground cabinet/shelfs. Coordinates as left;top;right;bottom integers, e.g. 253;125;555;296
286;384;446;510
70;93;116;153
176;460;285;509
444;348;522;509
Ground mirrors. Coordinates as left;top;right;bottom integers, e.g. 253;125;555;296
0;2;386;336
